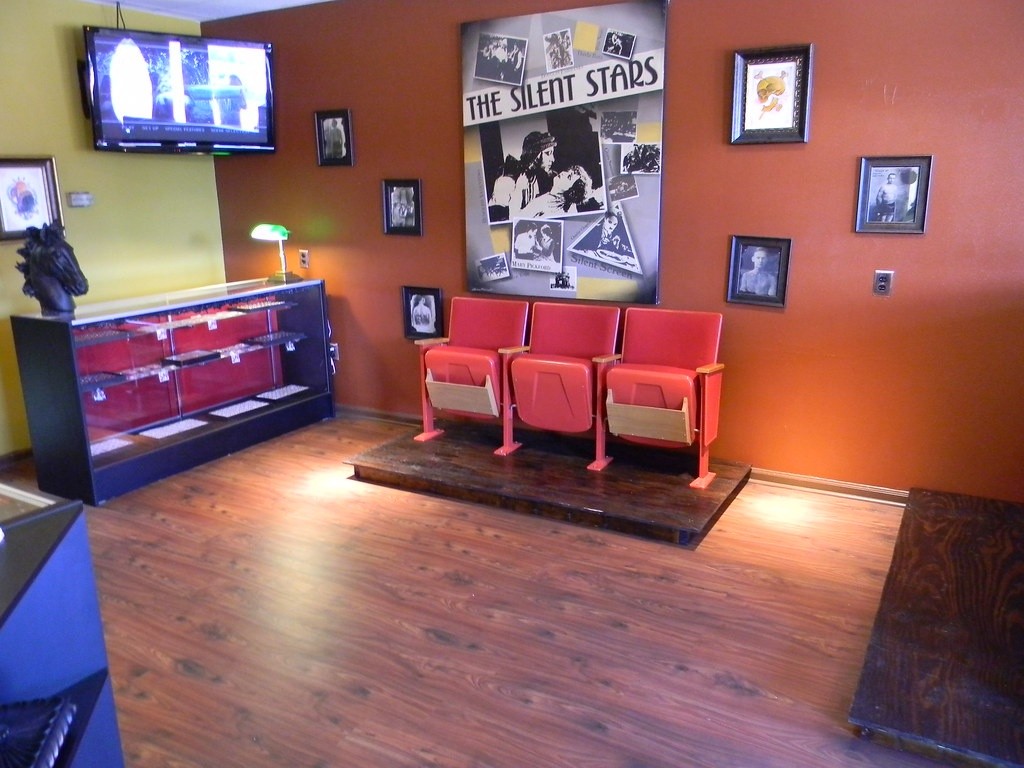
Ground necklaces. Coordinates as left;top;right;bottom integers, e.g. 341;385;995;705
544;239;551;242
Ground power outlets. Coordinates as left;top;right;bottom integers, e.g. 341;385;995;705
299;249;309;268
872;269;894;298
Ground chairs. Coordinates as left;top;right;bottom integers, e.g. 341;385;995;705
587;307;726;489
494;301;621;472
413;297;531;457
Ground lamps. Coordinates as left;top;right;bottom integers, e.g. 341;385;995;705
250;224;303;284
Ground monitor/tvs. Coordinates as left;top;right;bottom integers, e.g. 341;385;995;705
82;24;276;155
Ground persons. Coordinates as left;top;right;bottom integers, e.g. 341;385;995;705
551;34;572;69
413;295;431;326
605;32;623;55
876;173;897;222
488;131;593;221
741;247;777;296
331;119;343;157
394;203;414;227
579;208;626;255
214;75;246;127
623;144;660;172
515;223;556;263
555;272;570;287
483;39;523;72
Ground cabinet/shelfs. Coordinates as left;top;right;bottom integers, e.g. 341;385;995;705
10;277;337;507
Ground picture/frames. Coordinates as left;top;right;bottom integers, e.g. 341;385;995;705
725;234;792;309
855;153;933;234
729;41;815;145
313;109;354;167
381;178;423;237
0;153;67;241
401;285;445;339
